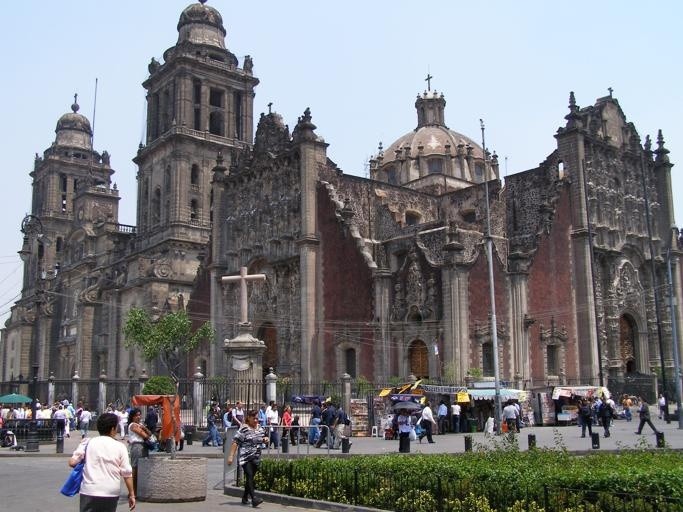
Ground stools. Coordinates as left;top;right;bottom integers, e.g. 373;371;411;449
371;424;377;436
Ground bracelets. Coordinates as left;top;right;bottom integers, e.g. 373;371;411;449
129;495;136;498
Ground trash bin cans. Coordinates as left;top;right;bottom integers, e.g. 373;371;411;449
467;418;478;433
399;430;410;453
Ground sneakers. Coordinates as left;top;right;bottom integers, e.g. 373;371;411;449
242;498;250;504
253;497;263;506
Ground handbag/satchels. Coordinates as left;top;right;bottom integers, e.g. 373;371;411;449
146;434;158;451
59;462;83;498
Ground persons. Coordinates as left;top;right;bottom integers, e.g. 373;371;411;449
127;408;152;498
622;394;633;421
69;413;136;512
634;397;658;434
228;409;270;506
658;393;666;419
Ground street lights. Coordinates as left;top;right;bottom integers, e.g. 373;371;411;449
664;222;682;430
14;210;58;451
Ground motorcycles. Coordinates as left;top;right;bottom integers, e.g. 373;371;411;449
0;419;16;450
